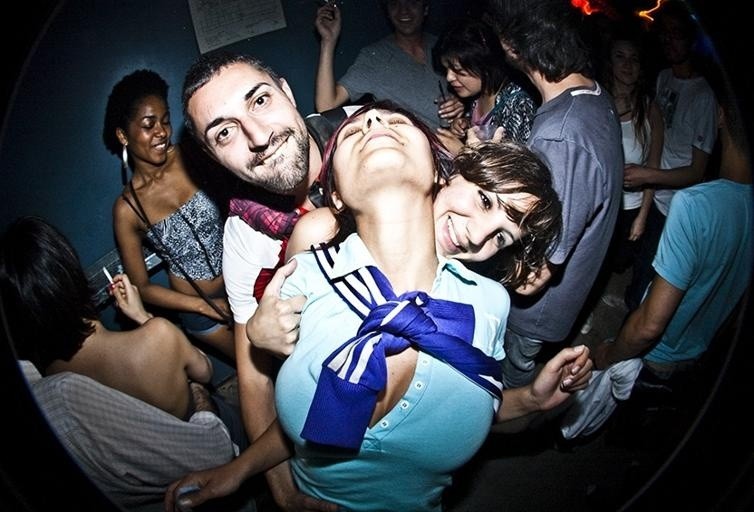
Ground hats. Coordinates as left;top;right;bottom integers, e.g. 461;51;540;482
308;102;440;209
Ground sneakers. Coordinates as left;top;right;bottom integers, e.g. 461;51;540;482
601;293;631;312
579;312;593;334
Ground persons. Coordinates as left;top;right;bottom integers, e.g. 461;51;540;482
100;1;754;511
4;214;252;512
